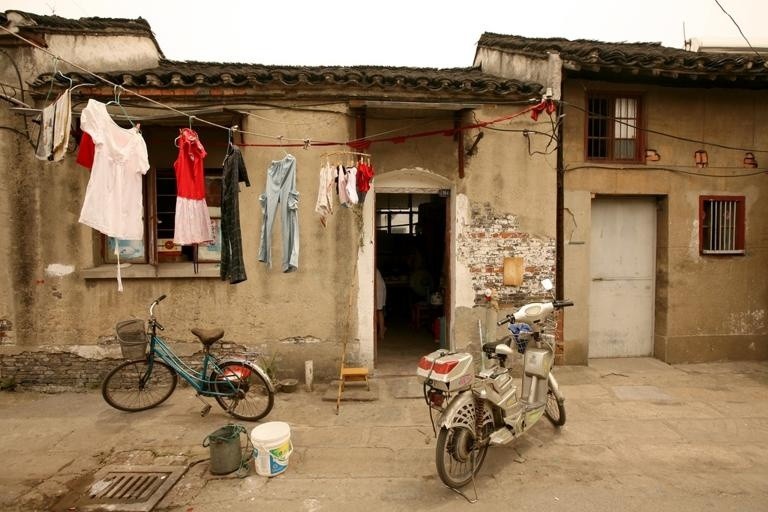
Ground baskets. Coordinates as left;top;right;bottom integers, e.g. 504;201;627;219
115;318;149;360
506;316;558;354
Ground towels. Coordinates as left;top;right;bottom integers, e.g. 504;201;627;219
33;99;55;162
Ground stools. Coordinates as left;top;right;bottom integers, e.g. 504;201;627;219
340;367;370;392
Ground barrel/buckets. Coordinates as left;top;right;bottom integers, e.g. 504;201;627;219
251;422;294;477
209;423;241;475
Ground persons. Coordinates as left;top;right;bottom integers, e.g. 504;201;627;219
376;267;386;340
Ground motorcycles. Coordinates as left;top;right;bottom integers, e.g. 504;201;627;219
416;278;574;504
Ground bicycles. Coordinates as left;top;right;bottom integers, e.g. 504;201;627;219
102;295;276;422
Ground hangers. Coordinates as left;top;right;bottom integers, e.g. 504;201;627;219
41;55;288;163
